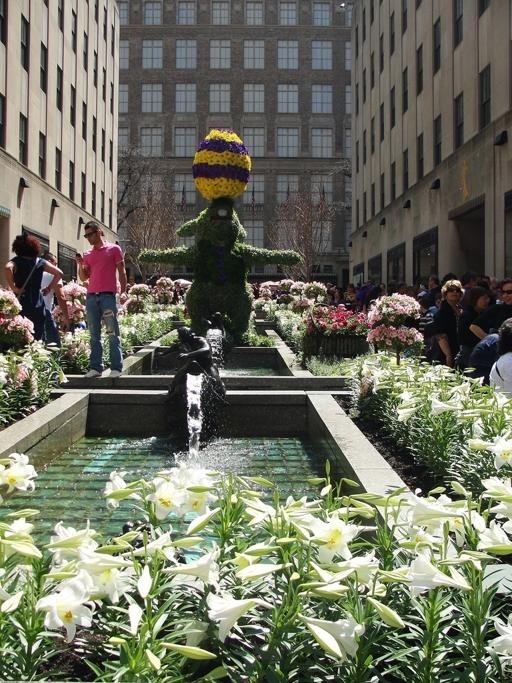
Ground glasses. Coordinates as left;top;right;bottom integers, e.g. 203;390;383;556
502;289;512;294
449;288;461;292
83;230;97;237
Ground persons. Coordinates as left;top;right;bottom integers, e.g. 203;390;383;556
206;311;225;330
332;270;512;391
166;324;227;409
4;232;65;342
75;220;129;380
40;250;70;348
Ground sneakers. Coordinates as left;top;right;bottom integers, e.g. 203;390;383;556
109;369;122;378
84;368;102;378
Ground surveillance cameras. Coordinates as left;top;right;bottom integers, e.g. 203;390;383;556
339;3;345;8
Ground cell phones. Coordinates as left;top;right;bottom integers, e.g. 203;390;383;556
76;253;81;257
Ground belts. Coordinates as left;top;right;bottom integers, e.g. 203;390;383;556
87;291;113;296
